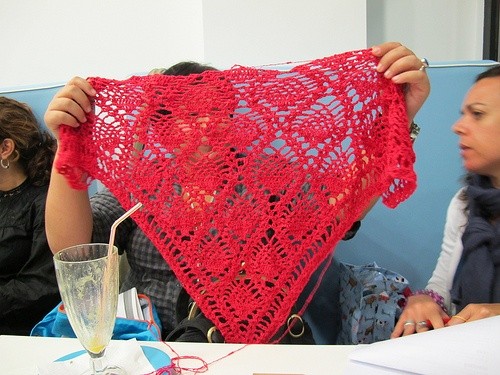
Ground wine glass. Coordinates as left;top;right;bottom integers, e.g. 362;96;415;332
53;243;126;375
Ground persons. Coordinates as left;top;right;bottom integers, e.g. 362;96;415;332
0;94;58;336
391;63;500;338
45;42;431;343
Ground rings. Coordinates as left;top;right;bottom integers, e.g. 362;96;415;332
416;321;427;326
452;316;467;322
404;320;414;326
419;58;429;71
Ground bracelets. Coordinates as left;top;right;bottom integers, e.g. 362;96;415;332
374;112;421;145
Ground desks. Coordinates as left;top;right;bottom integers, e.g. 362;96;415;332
0;335;355;375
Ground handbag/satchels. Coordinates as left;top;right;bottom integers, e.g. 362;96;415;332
30;286;162;341
163;212;346;344
341;264;409;345
